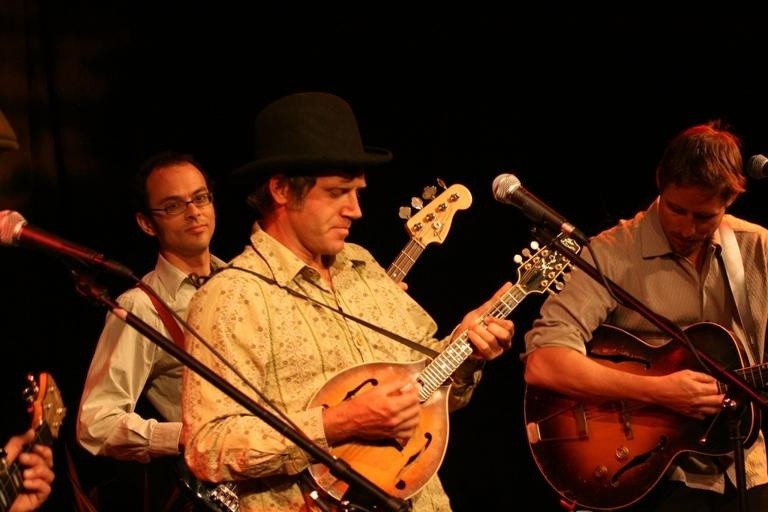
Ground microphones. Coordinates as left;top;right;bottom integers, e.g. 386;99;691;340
0;210;134;278
492;173;590;246
747;154;768;179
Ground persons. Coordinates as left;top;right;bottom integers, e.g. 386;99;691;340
75;153;229;464
0;109;56;512
180;89;515;512
519;124;768;511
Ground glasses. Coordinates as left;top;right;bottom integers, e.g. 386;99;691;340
147;193;213;215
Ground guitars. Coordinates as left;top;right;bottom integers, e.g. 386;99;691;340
524;320;768;511
0;371;68;512
177;176;473;512
